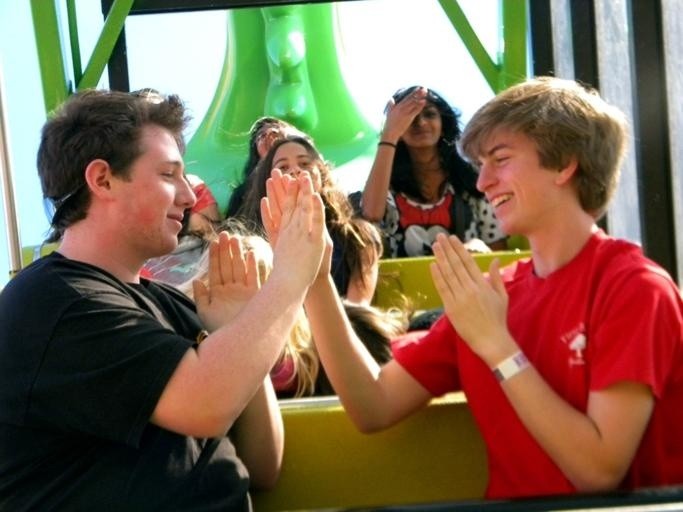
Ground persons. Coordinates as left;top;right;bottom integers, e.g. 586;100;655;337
124;87;225;243
1;82;329;512
172;232;393;402
259;75;682;502
359;82;513;261
236;134;385;308
225;116;314;222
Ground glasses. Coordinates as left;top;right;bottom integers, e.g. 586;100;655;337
42;183;84;223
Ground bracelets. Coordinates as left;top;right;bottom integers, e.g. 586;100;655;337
378;139;398;149
491;348;534;383
